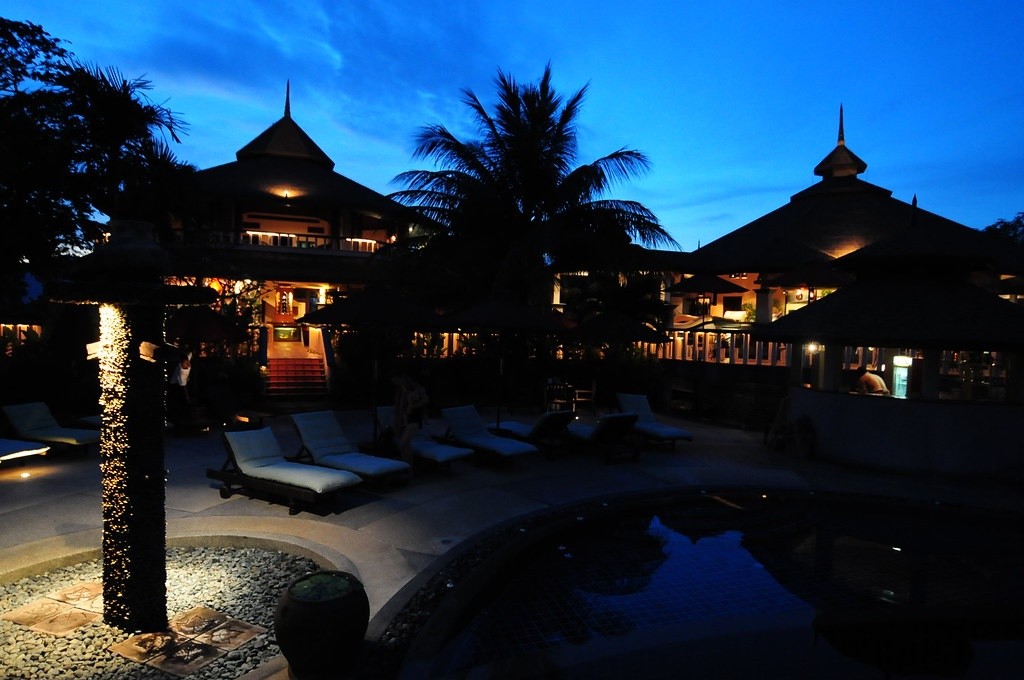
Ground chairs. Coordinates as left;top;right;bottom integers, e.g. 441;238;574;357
0;438;51;468
3;401;100;461
489;411;578;448
359;406;474;471
441;405;537;464
283;411;409;491
566;412;638;452
205;427;363;516
544;380;598;418
615;392;693;451
79;416;101;428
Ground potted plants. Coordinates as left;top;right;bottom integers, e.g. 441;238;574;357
781;414;816;459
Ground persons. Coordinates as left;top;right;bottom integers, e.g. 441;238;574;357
855;366;891;394
170;354;192;410
390;372;430;471
